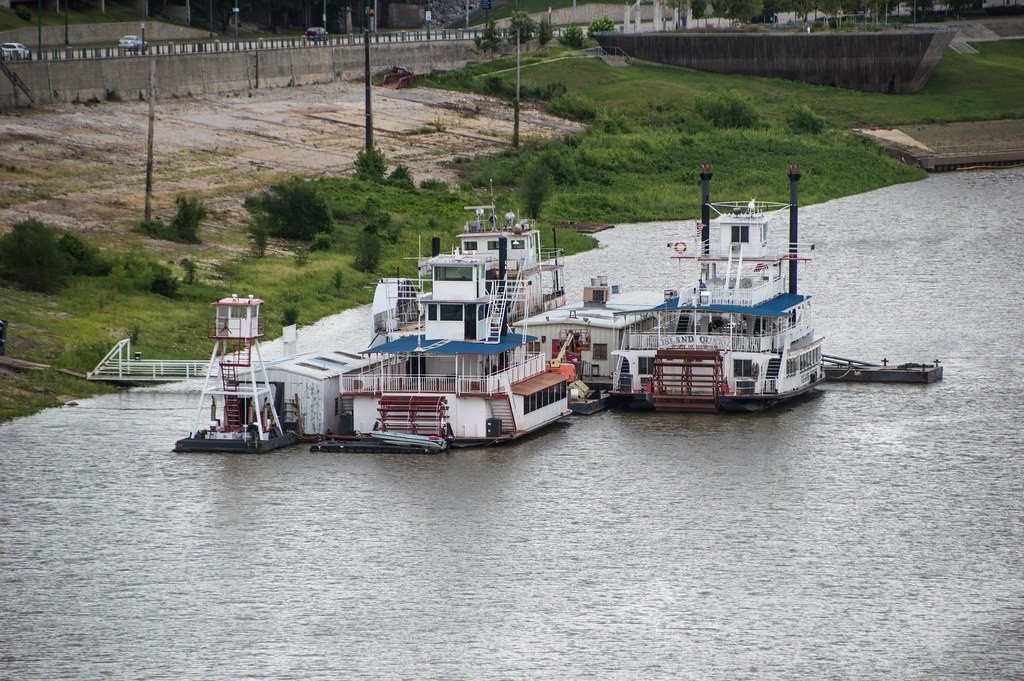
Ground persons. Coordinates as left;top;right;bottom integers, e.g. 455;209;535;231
698;278;707;306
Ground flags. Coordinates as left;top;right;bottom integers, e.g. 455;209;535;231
696;222;707;237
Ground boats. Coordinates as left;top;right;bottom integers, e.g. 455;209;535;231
604;162;826;417
174;181;577;457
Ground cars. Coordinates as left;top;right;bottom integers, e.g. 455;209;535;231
118;35;149;52
305;27;328;42
1;42;30;60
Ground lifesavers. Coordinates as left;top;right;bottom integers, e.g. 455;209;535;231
674;240;687;253
